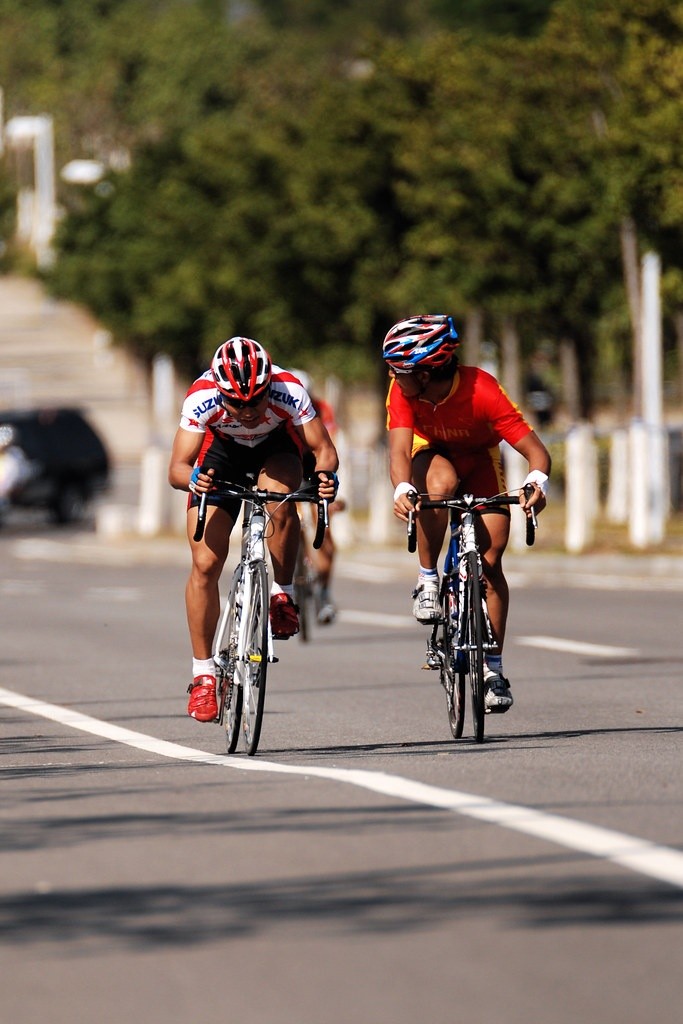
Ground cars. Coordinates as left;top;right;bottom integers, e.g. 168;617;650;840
0;405;110;522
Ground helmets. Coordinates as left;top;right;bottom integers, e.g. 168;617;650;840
212;337;272;401
383;315;460;369
289;369;313;395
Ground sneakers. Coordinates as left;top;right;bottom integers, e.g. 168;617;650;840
412;573;443;621
269;593;299;636
482;670;513;708
185;675;218;722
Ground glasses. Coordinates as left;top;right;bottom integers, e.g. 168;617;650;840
387;364;434;379
220;383;270;410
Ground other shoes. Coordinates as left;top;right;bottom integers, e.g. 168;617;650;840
314;590;335;624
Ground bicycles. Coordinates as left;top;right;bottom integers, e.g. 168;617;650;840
191;476;327;756
406;483;539;744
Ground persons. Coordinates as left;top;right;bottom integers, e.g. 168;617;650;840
382;313;553;706
281;365;337;626
168;335;340;720
